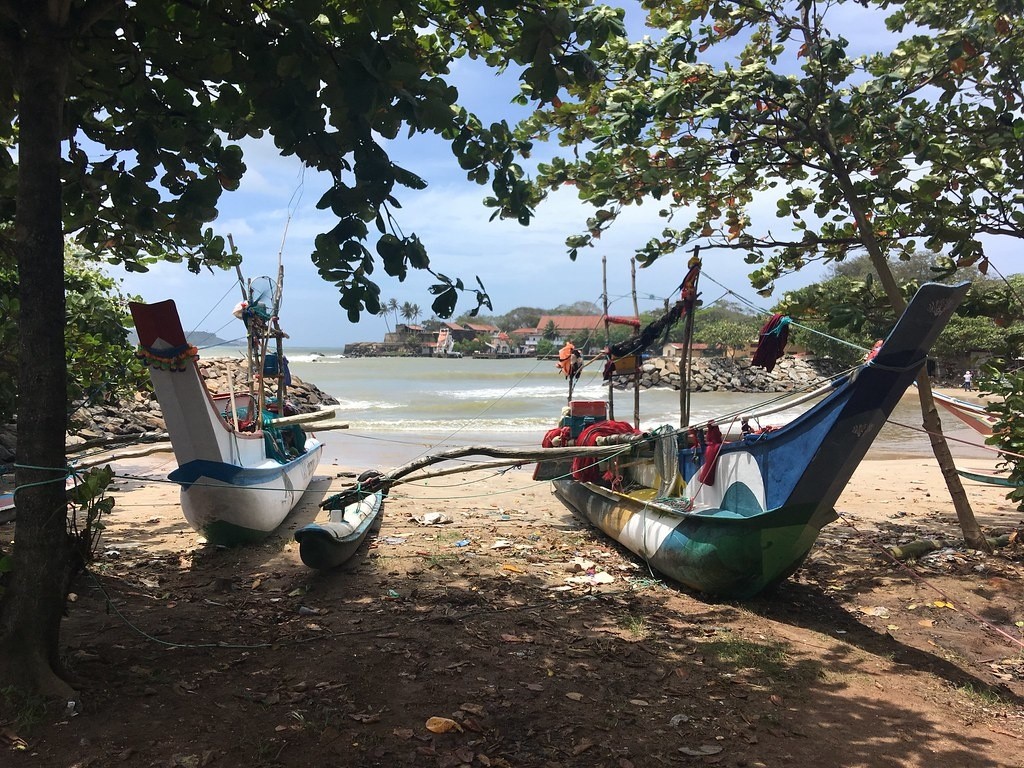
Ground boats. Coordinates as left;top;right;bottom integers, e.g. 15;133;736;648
292;486;386;571
126;230;354;550
533;242;974;600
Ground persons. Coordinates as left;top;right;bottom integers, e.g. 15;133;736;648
962;370;973;392
